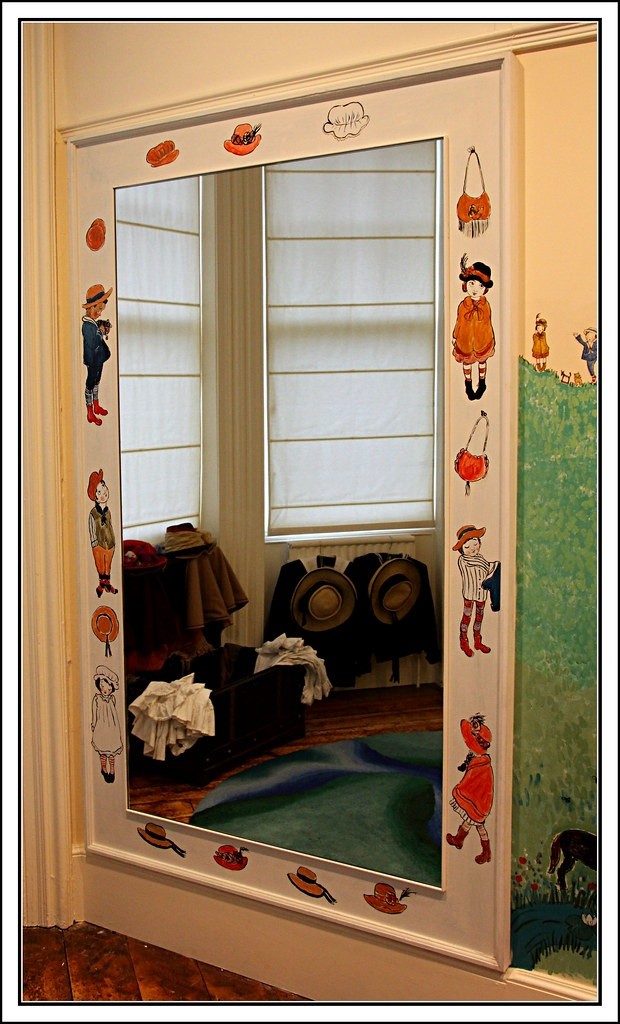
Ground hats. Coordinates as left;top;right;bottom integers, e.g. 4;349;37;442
291;567;357;632
123;540;167;570
167;523;216;559
367;558;421;625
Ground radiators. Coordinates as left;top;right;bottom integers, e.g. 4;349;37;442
286;533;416;573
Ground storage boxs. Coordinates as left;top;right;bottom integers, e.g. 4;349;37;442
126;642;307;790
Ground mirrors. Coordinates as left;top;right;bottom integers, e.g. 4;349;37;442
113;135;450;895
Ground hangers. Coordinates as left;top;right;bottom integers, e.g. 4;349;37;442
378;536;401;563
317;542;336;568
